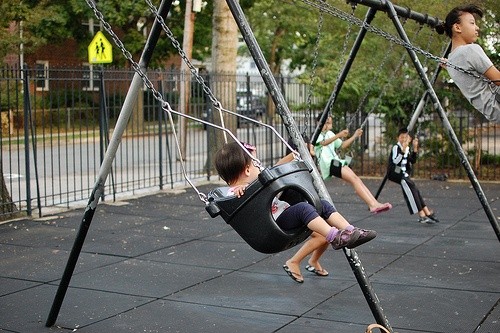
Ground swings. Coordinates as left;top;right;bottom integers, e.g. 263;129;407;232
328;18;423;179
301;0;500;96
85;0;321;256
387;28;435;185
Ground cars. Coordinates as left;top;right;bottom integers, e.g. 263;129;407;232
202;90;271;130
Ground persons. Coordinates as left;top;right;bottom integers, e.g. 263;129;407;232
393;128;439;225
311;113;392;213
215;136;377;283
435;5;500;123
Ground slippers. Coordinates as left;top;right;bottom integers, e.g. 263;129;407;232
283;265;304;282
305;264;329;276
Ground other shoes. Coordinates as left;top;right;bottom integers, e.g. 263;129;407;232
417;216;436;224
426;214;440;222
346;228;377;249
331;229;360;250
370;203;392;215
